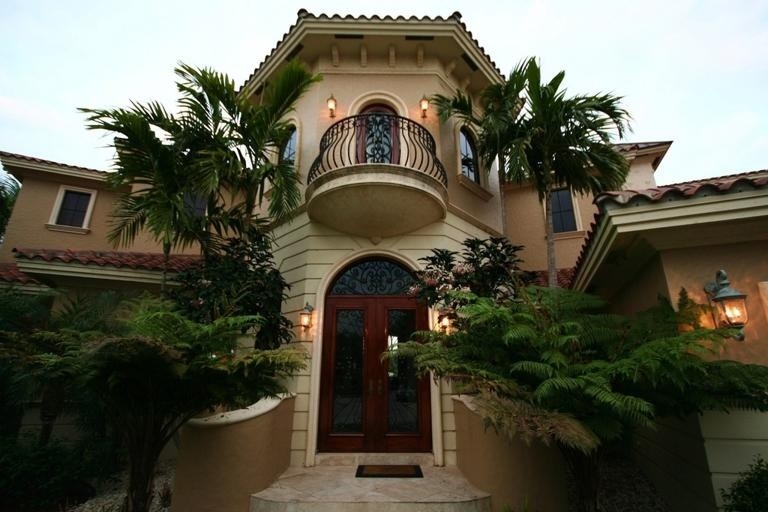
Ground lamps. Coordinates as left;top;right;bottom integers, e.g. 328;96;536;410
701;265;751;342
418;94;430;119
298;300;314;332
327;94;338;118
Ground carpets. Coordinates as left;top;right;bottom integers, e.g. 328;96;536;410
355;463;424;479
